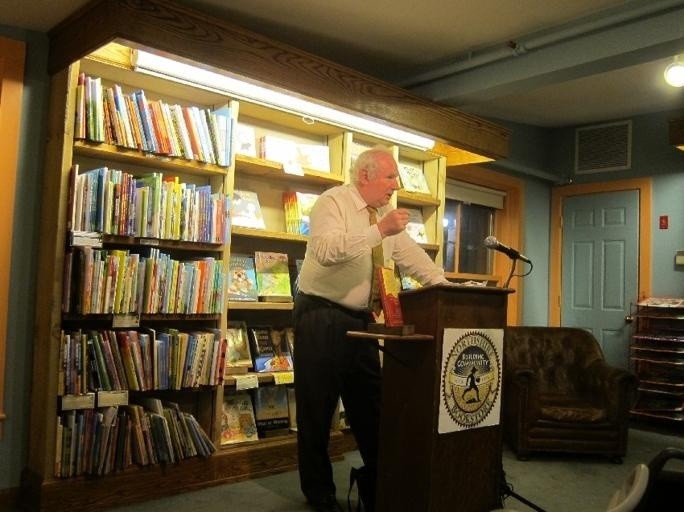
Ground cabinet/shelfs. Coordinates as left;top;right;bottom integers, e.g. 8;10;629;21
623;296;684;424
21;55;449;511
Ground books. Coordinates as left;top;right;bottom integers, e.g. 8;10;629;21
397;160;433;196
366;265;418;336
399;206;428;244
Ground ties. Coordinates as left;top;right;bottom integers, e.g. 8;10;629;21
367;205;385;317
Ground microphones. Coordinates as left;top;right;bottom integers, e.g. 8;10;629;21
483;234;532;264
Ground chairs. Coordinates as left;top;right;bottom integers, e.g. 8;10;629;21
502;325;642;466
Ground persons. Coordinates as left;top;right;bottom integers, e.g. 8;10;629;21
292;149;483;511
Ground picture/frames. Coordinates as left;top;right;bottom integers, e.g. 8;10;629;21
574;119;632;175
